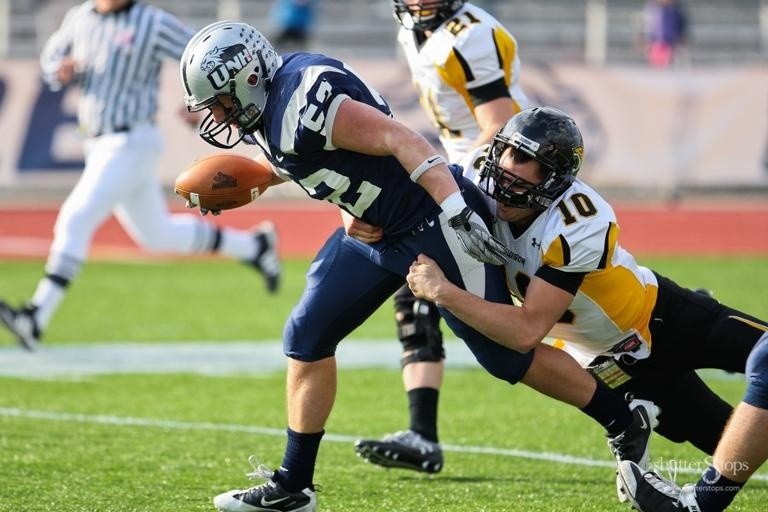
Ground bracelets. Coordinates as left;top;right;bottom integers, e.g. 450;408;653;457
438;189;468;220
409;152;448;184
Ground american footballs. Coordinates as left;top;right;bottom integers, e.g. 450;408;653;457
176;156;274;210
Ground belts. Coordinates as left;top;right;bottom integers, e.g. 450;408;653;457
87;124;128;138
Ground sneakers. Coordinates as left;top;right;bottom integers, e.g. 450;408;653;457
354;428;445;475
212;470;317;512
604;391;663;503
245;222;283;294
616;458;705;512
0;301;43;352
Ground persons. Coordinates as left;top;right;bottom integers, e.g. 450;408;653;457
259;0;318;56
1;0;282;354
353;0;531;475
404;107;768;462
613;330;768;511
636;0;688;73
177;18;659;512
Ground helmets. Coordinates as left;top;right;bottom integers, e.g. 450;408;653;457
492;105;585;214
180;19;285;135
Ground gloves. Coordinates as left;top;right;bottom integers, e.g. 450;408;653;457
446;204;510;267
176;191;222;218
